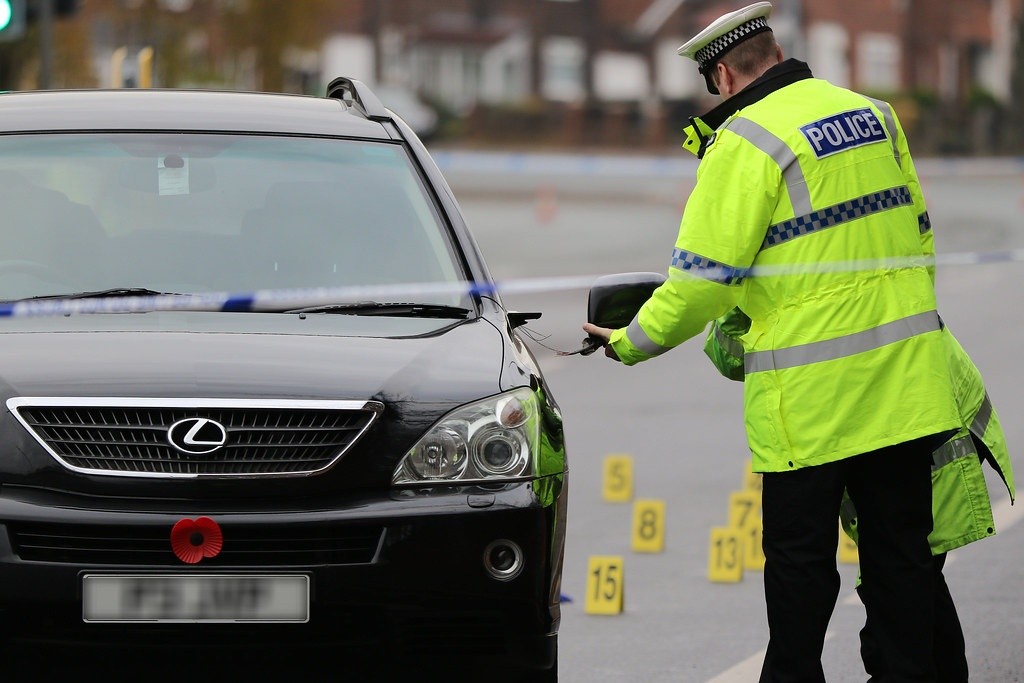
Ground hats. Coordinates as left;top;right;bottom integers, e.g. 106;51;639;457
674;1;774;95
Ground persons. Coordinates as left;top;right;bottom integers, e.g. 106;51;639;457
703;307;1016;683
584;0;961;683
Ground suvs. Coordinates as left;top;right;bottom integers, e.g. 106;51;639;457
0;71;670;683
